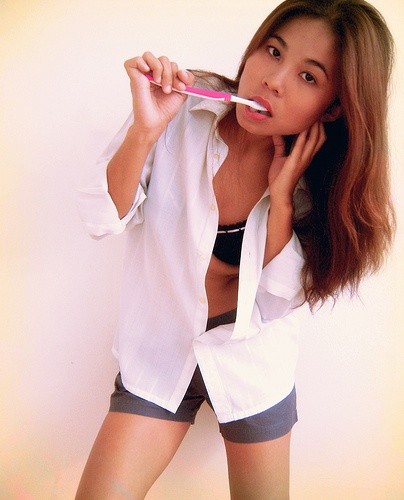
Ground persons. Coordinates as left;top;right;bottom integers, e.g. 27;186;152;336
76;0;399;495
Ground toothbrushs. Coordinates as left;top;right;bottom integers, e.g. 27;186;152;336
141;73;266;111
